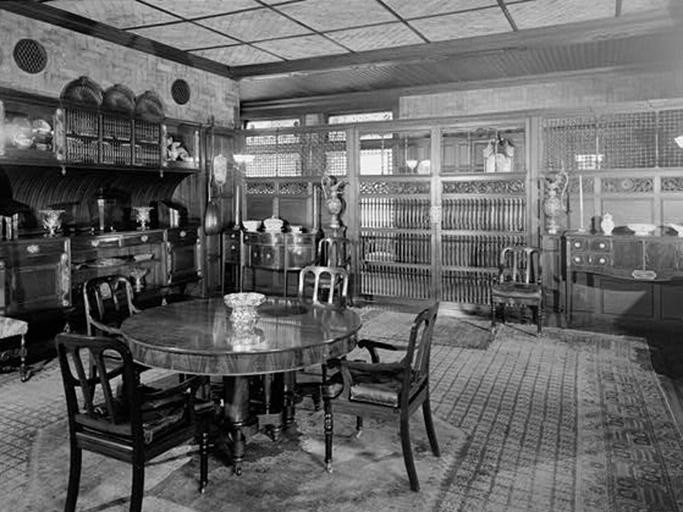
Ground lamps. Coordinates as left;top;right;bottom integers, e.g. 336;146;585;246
232;154;255;171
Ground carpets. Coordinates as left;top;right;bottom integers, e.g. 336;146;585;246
357;312;495;351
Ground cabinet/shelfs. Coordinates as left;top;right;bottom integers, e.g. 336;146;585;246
55;238;316;316
0;90;201;172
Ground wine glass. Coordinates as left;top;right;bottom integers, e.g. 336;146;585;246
133;206;155;230
38;210;66;238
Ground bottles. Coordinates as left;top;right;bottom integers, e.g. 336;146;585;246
600;212;614;235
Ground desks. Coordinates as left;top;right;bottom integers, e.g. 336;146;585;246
121;296;364;478
563;232;682;325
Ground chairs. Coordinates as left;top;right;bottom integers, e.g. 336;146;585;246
305;238;352;303
82;274;185;411
0;315;29;382
491;245;544;334
319;303;438;493
298;265;349;306
55;332;215;510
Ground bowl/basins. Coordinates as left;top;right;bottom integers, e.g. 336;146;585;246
223;292;267;308
627;223;656;236
243;219;302;233
669;223;683;236
